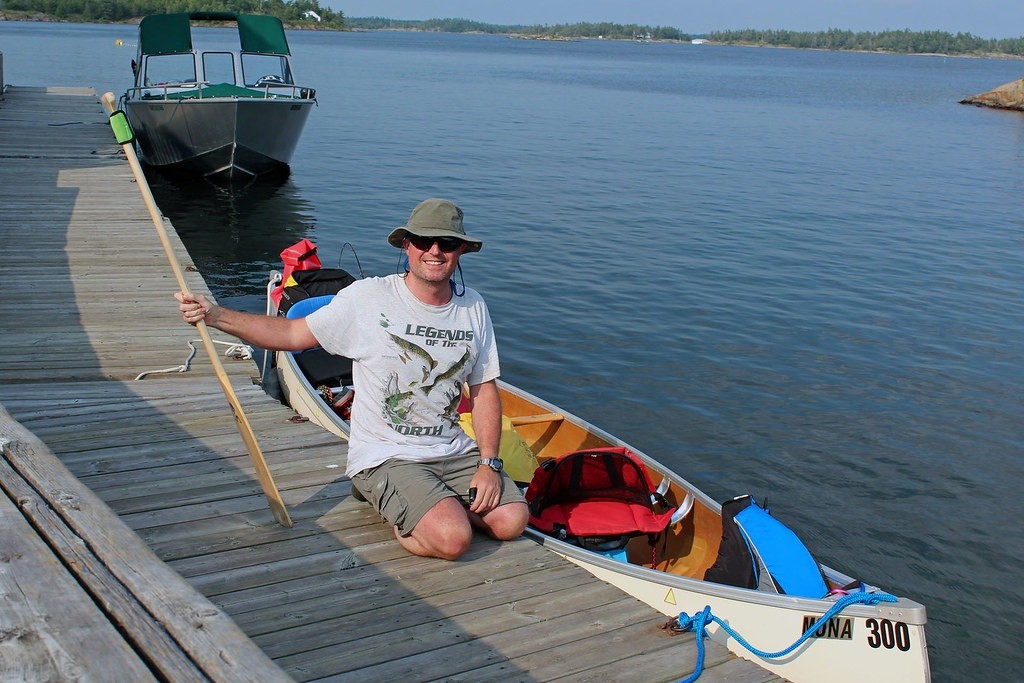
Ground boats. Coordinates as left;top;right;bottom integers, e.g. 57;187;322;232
260;242;932;683
121;11;317;187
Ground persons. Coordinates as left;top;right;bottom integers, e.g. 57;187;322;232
175;199;528;560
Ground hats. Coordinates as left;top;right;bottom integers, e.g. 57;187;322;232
387;198;484;254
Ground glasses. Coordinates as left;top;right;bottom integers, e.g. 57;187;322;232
404;233;463;251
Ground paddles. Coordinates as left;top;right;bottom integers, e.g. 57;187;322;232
101;91;295;531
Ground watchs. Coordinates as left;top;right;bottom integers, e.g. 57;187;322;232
477;457;503;472
